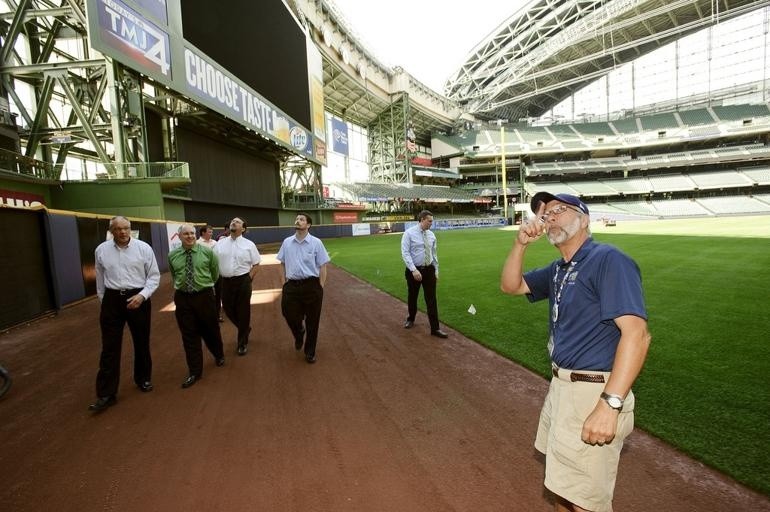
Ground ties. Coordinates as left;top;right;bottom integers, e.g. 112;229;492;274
185;250;193;292
422;231;430;266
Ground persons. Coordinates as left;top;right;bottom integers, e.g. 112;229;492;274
496;191;653;512
86;213;163;411
399;210;449;341
216;233;229;242
194;225;226;322
167;222;224;388
275;213;329;365
225;220;232;237
212;216;263;356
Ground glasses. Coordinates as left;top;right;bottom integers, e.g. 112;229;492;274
542;205;583;214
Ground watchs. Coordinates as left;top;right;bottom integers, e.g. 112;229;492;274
599;392;625;415
411;268;416;273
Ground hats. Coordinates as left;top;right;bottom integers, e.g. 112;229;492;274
531;192;589;215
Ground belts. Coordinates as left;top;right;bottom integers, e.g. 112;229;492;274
552;366;604;384
106;288;140;296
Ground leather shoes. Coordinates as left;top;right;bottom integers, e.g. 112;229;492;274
237;327;251;356
89;396;117;410
138;381;152;392
181;376;202;387
405;320;413;328
295;328;305;351
306;350;316;362
215;354;224;367
431;328;448;338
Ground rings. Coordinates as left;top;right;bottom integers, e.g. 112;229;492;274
598;439;605;444
